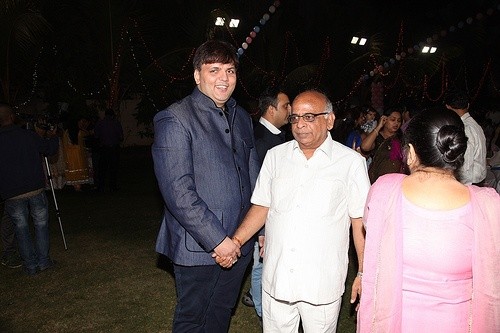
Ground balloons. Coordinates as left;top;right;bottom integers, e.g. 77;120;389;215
235;0;279;59
360;4;500;81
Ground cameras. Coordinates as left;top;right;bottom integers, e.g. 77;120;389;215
26;122;38;129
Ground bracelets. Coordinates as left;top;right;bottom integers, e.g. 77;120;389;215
232;235;242;248
357;272;363;278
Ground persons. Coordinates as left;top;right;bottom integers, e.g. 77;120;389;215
243;84;499;320
212;90;372;333
356;108;500;333
62;117;90;191
0;104;59;274
151;39;265;333
91;108;125;193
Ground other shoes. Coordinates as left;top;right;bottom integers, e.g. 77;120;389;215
242;288;254;306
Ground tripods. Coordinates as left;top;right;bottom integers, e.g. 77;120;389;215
8;152;68;251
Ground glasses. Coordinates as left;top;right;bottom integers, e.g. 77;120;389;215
287;113;330;124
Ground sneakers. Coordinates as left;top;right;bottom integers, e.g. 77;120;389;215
1;256;22;268
